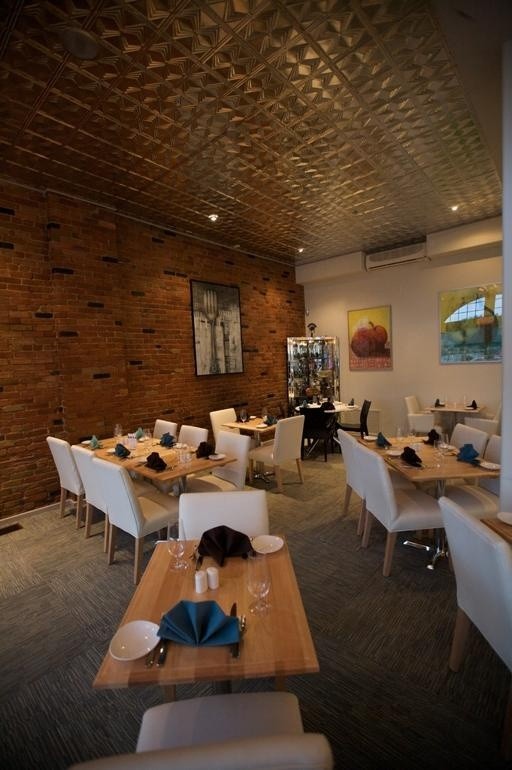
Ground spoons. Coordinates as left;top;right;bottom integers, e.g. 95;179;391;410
238;615;248;635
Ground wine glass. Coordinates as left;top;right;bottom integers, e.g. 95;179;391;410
171;432;182;468
432;432;449;472
239;408;248;427
165;519;189;573
246;551;271;616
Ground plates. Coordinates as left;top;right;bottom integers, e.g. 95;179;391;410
256;424;268;428
80;440;92;446
251;535;284;554
478;460;502;472
347;406;356;409
385;449;406;457
249;415;256;420
363;435;377;441
107;620;162;662
208;454;225;461
106;446;115;455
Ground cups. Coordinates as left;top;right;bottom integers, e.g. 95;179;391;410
128;433;137;450
181;442;193;463
194;569;208;594
207;566;219;591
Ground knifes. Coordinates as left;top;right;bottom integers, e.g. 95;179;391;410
229;603;238;655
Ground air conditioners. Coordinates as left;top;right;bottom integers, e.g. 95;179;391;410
364;242;427;272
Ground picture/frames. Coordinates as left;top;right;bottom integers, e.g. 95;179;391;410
348;305;394;372
189;279;244;378
437;282;504;367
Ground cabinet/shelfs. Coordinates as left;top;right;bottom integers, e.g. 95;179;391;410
286;336;341;415
